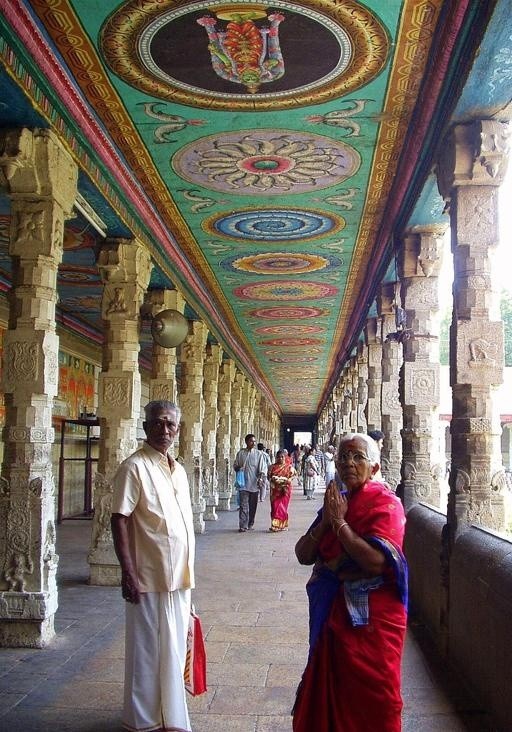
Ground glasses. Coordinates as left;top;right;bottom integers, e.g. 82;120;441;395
333;453;369;464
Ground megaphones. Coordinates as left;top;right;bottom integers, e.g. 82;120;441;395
151;308;191;348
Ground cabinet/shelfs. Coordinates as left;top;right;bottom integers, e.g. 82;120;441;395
56;417;101;524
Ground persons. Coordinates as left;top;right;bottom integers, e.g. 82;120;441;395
367;429;386;482
267;450;299;532
235;443;339;510
109;399;197;732
290;432;410;732
233;434;267;532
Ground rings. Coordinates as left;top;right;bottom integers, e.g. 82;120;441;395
126;597;130;599
331;497;334;500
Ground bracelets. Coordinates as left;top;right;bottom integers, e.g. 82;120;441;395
336;522;348;536
309;532;320;543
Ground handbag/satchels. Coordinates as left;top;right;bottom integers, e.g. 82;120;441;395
184;606;205;696
236;471;245;488
307;470;315;477
